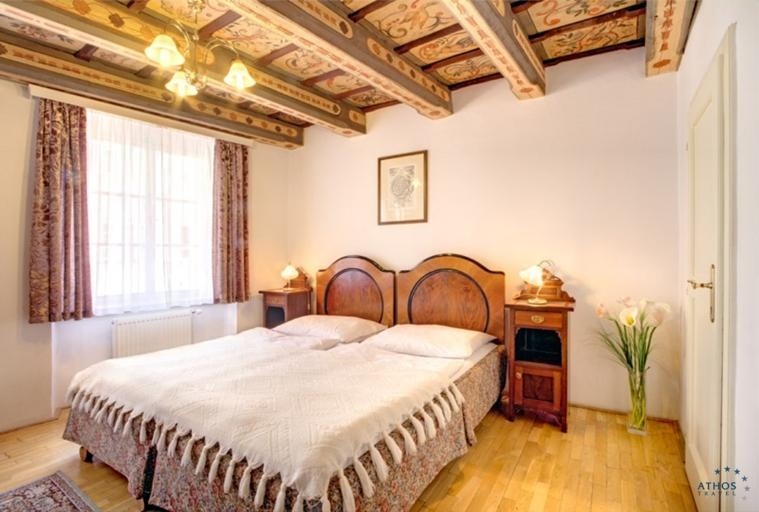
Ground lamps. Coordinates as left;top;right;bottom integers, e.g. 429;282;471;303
281;262;299;290
519;259;555;304
144;1;258;97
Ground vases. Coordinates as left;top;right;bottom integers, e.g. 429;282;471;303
625;368;649;437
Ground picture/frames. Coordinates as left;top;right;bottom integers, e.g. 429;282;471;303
376;149;429;225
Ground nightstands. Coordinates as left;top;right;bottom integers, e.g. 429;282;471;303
258;268;313;330
499;268;575;433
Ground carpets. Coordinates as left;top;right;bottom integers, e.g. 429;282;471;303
0;469;98;512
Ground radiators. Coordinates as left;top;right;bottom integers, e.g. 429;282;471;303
109;308;201;360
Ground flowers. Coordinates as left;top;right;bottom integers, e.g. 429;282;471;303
593;296;668;429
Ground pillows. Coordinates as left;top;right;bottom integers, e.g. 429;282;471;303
269;312;389;343
362;322;498;358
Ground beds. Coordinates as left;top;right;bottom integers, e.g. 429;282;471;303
60;252;506;512
